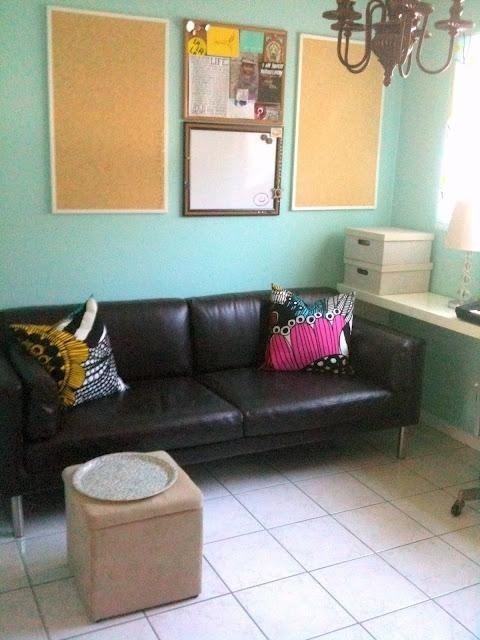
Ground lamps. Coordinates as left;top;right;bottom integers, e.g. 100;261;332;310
320;1;479;90
441;203;479;310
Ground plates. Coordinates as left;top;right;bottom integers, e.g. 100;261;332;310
72;451;179;504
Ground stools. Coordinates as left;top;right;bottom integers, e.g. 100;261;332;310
59;446;206;625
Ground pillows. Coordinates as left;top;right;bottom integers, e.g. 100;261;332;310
6;291;136;415
259;285;359;381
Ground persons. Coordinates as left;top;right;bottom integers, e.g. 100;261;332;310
233;52;258;100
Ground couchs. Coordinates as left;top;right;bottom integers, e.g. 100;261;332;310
0;283;429;537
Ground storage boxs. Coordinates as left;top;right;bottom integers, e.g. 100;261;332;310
341;225;434;298
